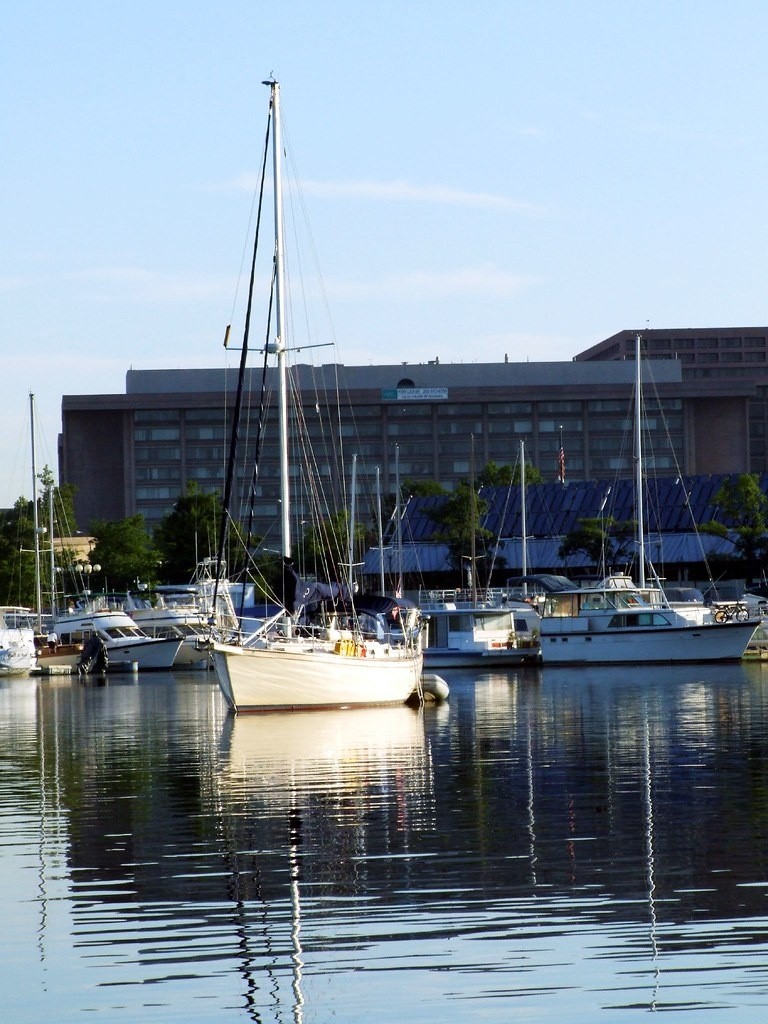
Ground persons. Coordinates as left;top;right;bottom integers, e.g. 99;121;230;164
47;630;57;653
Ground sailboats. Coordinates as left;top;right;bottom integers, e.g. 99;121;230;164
0;77;768;713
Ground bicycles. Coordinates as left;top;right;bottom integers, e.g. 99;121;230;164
714;599;750;623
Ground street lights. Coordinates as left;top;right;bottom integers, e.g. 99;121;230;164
75;563;101;600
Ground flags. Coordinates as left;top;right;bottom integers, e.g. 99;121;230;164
557;437;566;483
